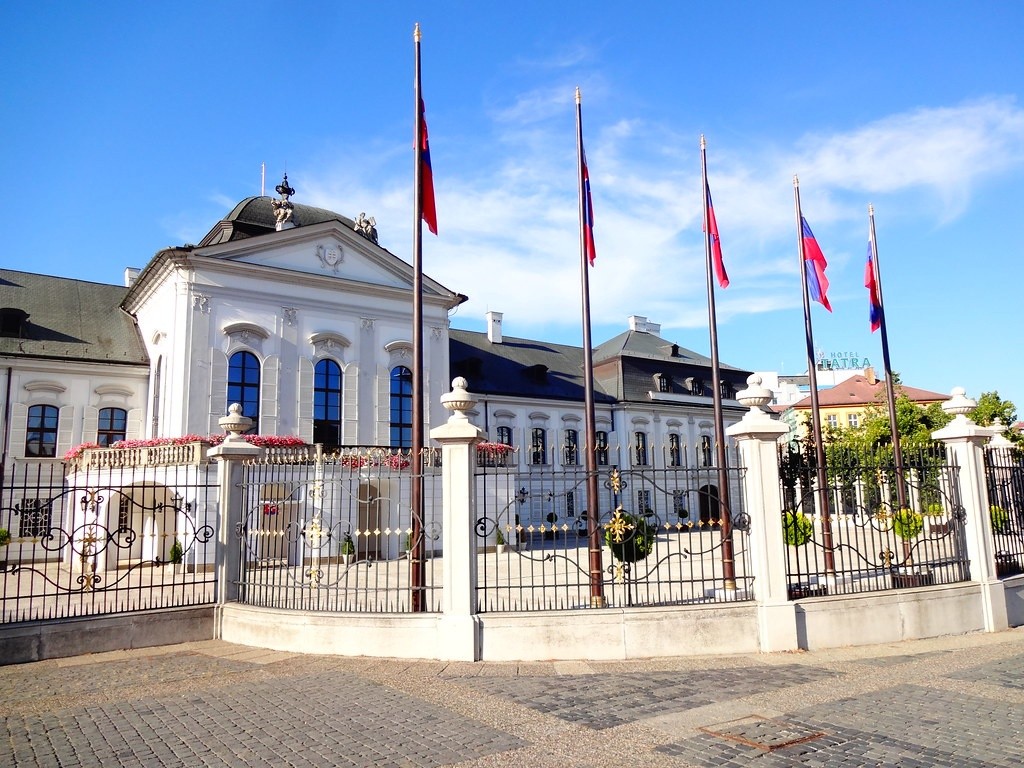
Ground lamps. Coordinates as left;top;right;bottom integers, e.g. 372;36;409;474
515;487;529;506
170;490;191;513
81;497;88;512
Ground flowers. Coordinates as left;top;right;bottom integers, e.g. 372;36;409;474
701;515;720;528
64;434;305;462
338;455;365;470
476;442;515;454
264;504;279;516
387;452;410;470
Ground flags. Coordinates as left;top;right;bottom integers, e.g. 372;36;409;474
582;148;596;268
865;239;882;334
703;179;729;289
801;216;832;313
420;100;437;235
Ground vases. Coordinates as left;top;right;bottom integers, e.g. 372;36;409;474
702;525;719;531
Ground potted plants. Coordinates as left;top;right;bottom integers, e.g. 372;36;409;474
495;529;505;554
342;534;355;564
678;509;689;532
405;535;412;560
518;528;527;550
578;510;588;537
644;508;655;533
890;509;933;589
782;510;827;599
991;504;1019;575
544;513;560;540
169;540;182;574
0;529;12;570
928;503;948;533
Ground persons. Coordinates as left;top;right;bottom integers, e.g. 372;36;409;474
354;211;377;244
271;198;292;224
